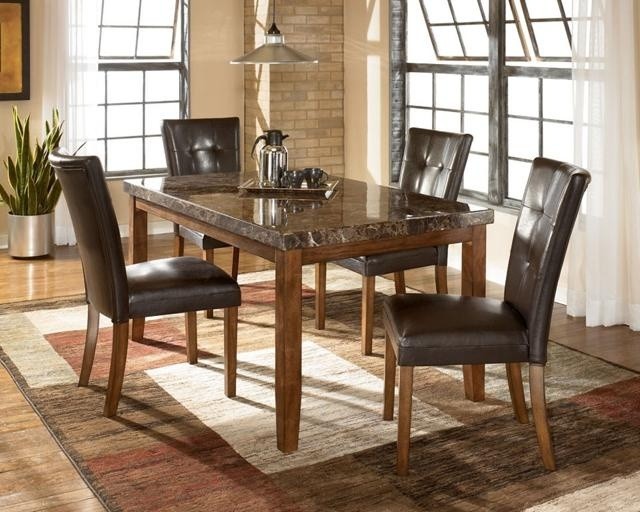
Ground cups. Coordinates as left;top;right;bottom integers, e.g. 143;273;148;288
304;167;329;188
279;170;303;189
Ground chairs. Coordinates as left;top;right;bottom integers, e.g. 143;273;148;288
48;151;242;419
161;118;241;321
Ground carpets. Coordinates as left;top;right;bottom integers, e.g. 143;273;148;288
0;259;639;510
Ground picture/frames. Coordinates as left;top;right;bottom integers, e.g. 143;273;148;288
0;1;30;101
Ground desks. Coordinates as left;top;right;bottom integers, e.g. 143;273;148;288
121;170;495;455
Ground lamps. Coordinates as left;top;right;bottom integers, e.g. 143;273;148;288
229;0;320;64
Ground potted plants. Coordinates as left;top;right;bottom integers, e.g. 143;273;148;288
2;105;88;258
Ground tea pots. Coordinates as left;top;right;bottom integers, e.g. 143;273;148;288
251;129;290;187
